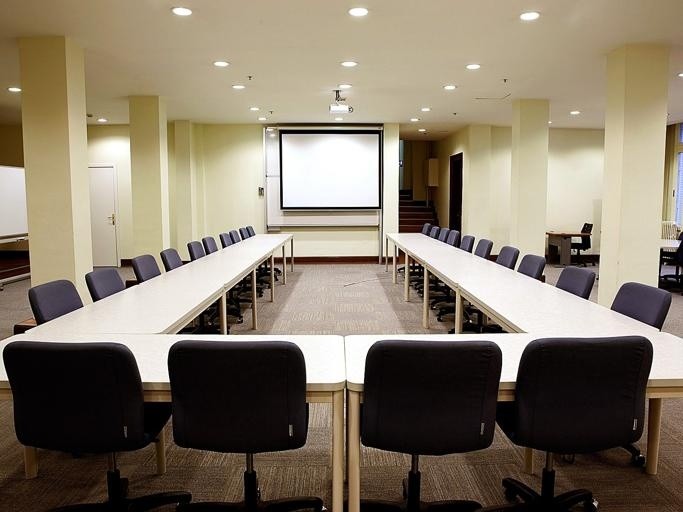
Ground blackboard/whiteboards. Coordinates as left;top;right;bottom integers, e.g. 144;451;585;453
264;126;383;227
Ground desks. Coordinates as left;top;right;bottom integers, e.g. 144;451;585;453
382;233;659;336
0;333;343;512
345;330;683;511
1;233;294;482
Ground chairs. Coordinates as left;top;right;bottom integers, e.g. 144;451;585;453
360;340;503;512
2;339;189;512
26;225;281;336
485;333;654;511
164;339;318;512
398;223;673;469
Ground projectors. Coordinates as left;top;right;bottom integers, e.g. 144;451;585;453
329;105;353;113
